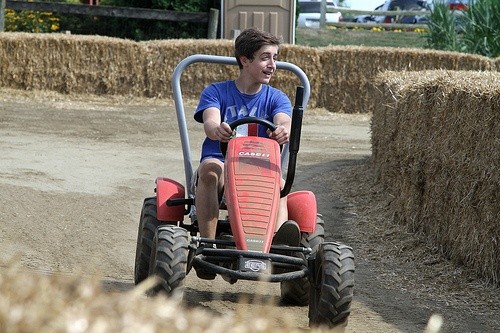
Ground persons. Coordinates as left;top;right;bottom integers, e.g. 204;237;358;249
191;27;301;279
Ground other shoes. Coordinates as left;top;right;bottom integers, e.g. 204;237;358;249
269;220;302;256
196;244;218;280
